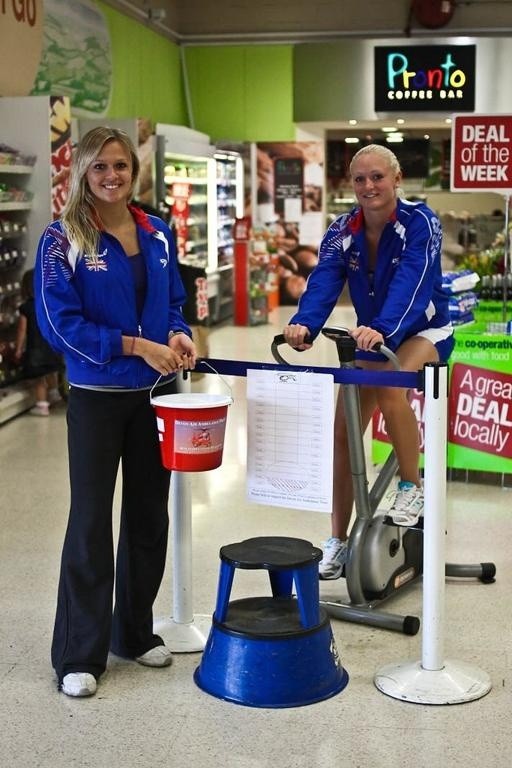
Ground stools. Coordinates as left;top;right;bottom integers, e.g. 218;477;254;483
216;533;322;629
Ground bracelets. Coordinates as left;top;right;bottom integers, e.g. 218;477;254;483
168;330;184;339
131;336;135;357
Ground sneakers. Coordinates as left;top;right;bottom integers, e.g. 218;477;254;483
137;645;172;668
61;672;97;697
382;481;424;527
318;537;349;579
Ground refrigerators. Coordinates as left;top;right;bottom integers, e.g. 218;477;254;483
215;148;245;326
163;136;215;327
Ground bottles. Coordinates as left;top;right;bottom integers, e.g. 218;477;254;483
488;323;512;335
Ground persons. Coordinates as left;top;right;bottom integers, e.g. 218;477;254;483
13;269;66;417
283;143;457;581
34;126;197;698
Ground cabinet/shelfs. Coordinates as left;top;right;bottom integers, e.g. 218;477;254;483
158;151;246;328
0;165;50;425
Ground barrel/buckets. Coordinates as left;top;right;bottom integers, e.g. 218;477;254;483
149;359;233;471
